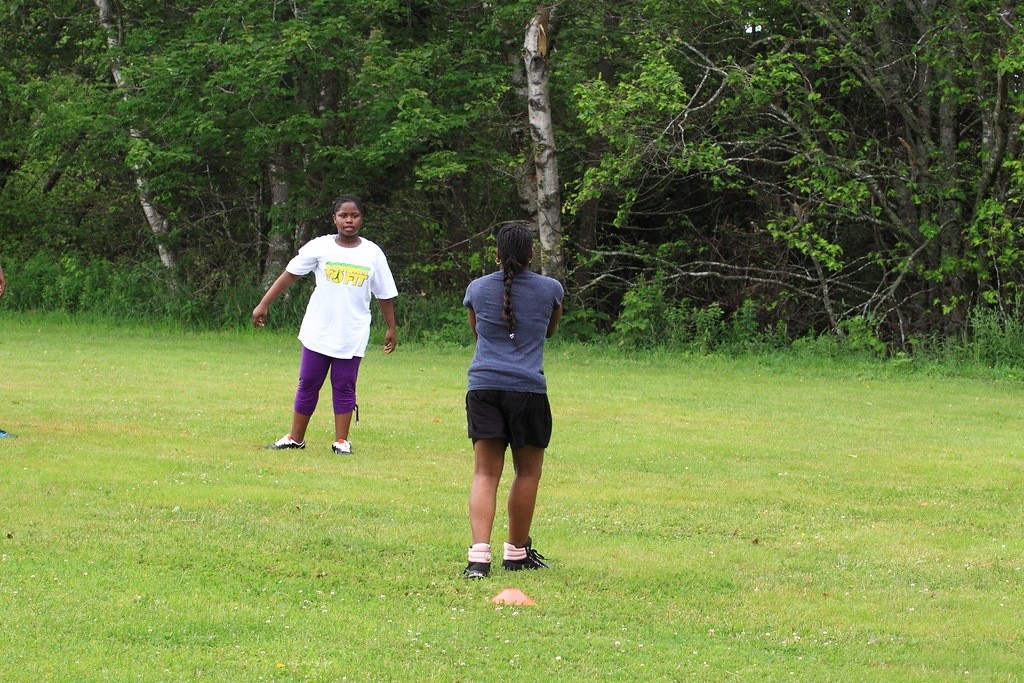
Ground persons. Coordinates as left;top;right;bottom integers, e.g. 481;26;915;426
463;223;564;582
252;194;399;455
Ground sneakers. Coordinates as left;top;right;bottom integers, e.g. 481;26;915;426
463;542;491;581
265;433;306;451
0;429;18;439
332;438;354;455
502;535;552;572
